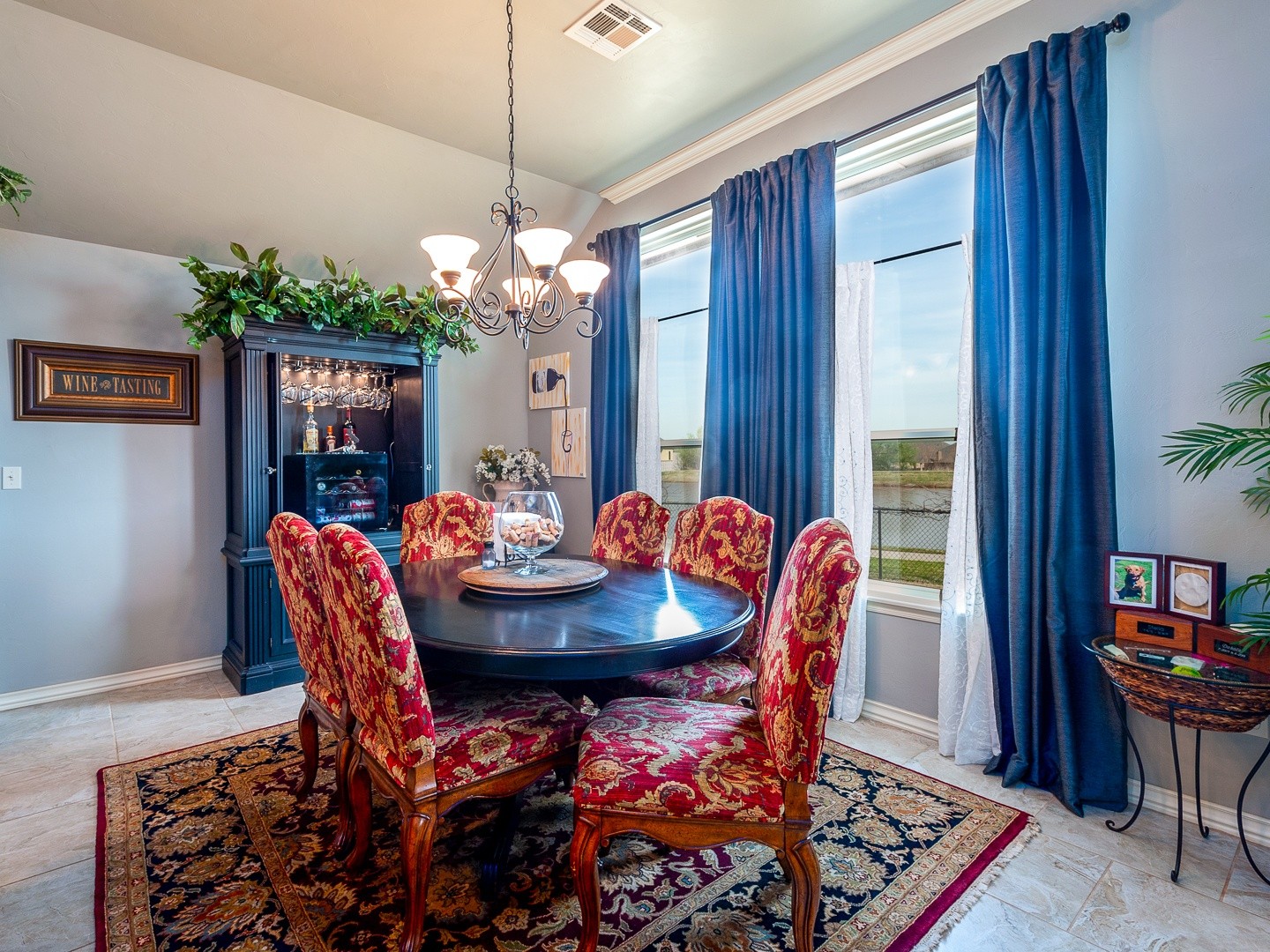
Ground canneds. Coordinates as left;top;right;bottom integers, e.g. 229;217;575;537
348;499;375;511
317;482;326;491
339;482;357;491
317;508;375;523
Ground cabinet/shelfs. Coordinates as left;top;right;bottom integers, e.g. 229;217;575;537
218;313;450;697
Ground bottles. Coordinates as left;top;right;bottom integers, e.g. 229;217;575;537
303;401;319;454
343;405;356;447
323;425;336;452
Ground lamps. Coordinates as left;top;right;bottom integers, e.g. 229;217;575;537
419;0;613;351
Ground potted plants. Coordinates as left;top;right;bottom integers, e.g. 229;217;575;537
174;240;481;365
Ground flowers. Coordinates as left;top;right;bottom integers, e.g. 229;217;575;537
475;444;552;488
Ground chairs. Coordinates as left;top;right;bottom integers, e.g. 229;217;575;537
552;490;672;707
399;490;497;563
309;521;591;952
265;511;499;859
586;494;777;720
568;514;859;952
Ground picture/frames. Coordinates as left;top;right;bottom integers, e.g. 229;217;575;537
1103;550;1164;614
13;338;201;427
1163;554;1228;627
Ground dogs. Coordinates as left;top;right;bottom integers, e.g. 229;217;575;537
1123;564;1150;603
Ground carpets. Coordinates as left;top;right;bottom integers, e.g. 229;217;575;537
90;713;1042;952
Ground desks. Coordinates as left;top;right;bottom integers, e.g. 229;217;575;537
384;552;758;904
1078;631;1270;886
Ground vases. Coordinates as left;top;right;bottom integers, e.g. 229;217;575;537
481;472;535;513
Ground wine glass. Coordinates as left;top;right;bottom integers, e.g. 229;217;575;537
499;491;566;576
281;363;391;410
